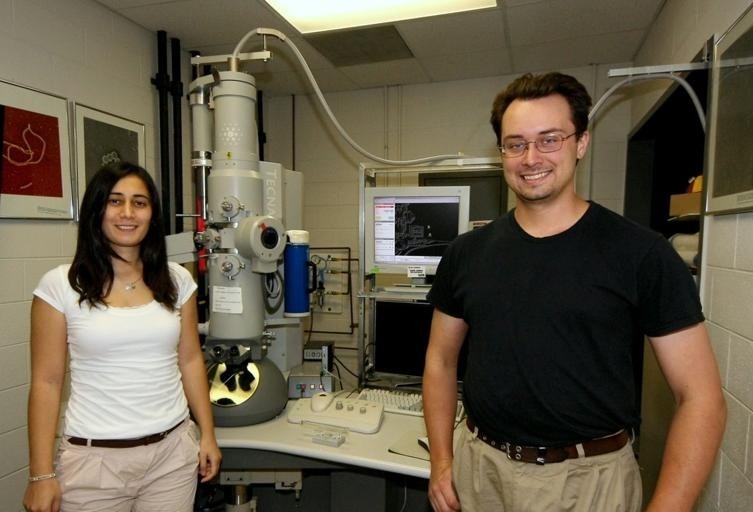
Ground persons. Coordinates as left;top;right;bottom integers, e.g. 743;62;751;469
21;160;222;512
420;69;729;512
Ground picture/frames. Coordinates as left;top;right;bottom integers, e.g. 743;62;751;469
71;101;146;224
0;78;74;224
699;1;753;216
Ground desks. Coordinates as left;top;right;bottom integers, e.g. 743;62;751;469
187;384;473;512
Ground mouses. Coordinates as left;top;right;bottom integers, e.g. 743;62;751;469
418;436;430;453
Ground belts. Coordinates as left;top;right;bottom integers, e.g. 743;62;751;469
466;418;627;465
68;420;184;448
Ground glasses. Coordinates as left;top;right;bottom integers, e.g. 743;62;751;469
496;133;576;158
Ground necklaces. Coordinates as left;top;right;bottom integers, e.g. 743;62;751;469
110;270;146;291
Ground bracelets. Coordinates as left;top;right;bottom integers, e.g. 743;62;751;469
28;469;60;483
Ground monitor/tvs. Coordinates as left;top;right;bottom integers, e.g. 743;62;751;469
367;292;468;397
364;185;471;275
418;168;509;229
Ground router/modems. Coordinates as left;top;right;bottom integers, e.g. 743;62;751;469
321;341;334;373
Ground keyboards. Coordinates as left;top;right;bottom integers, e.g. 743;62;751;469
355;389;465;422
